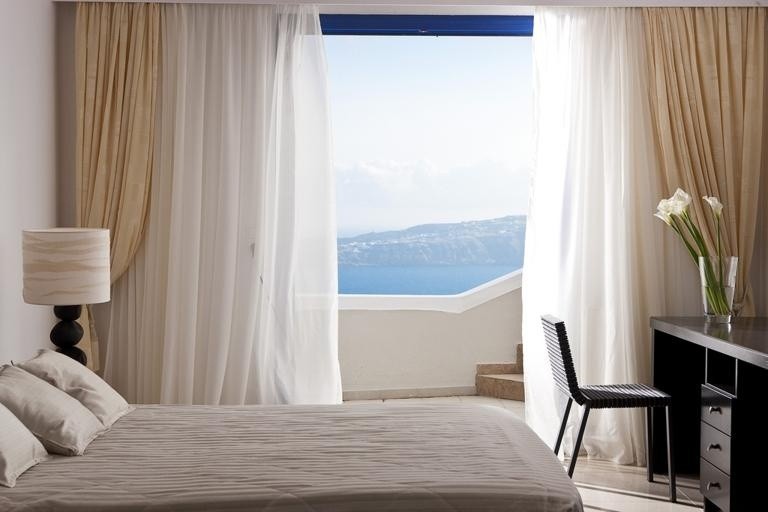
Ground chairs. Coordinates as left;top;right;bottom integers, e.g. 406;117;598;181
539;311;678;504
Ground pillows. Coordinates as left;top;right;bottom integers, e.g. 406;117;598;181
0;347;135;487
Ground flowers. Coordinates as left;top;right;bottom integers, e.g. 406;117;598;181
653;187;733;316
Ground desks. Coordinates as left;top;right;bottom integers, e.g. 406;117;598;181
648;316;768;511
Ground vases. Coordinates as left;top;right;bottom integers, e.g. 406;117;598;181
696;255;738;325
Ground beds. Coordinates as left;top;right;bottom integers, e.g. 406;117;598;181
1;400;582;512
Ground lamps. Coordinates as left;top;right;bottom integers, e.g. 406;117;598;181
17;226;112;367
